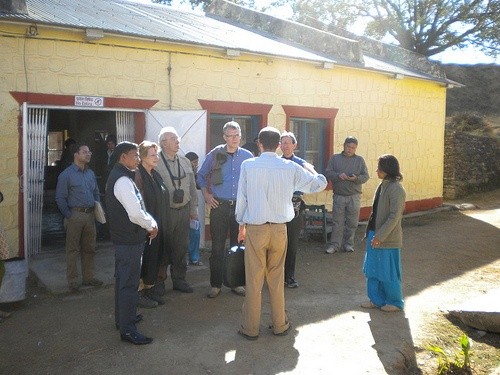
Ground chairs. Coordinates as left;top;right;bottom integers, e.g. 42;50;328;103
303;204;333;243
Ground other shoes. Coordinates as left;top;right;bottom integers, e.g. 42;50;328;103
381;304;400;312
176;284;193;292
208;287;220;297
192;261;203;266
232;286;245;294
69;282;79;288
137;291;158;307
361;301;380;308
325;247;336;253
84;278;103;286
345;245;354;252
0;310;12;318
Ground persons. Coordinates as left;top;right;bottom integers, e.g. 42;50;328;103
325;135;369;254
152;127;198;292
197;120;255;297
60;134;115;179
104;141;154;345
0;192;11;318
135;141;169;308
184;152;204;265
56;143;103;290
362;155;406;311
235;126;328;341
280;132;308;288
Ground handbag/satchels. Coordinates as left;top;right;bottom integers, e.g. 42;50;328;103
224;242;245;286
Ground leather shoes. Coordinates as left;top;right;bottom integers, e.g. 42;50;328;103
115;314;143;329
121;331;152;345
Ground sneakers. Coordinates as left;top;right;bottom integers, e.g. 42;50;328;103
285;277;299;288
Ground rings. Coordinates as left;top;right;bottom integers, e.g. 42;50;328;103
376;243;378;244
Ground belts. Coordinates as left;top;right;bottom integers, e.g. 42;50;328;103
217;199;236;206
80;208;93;213
